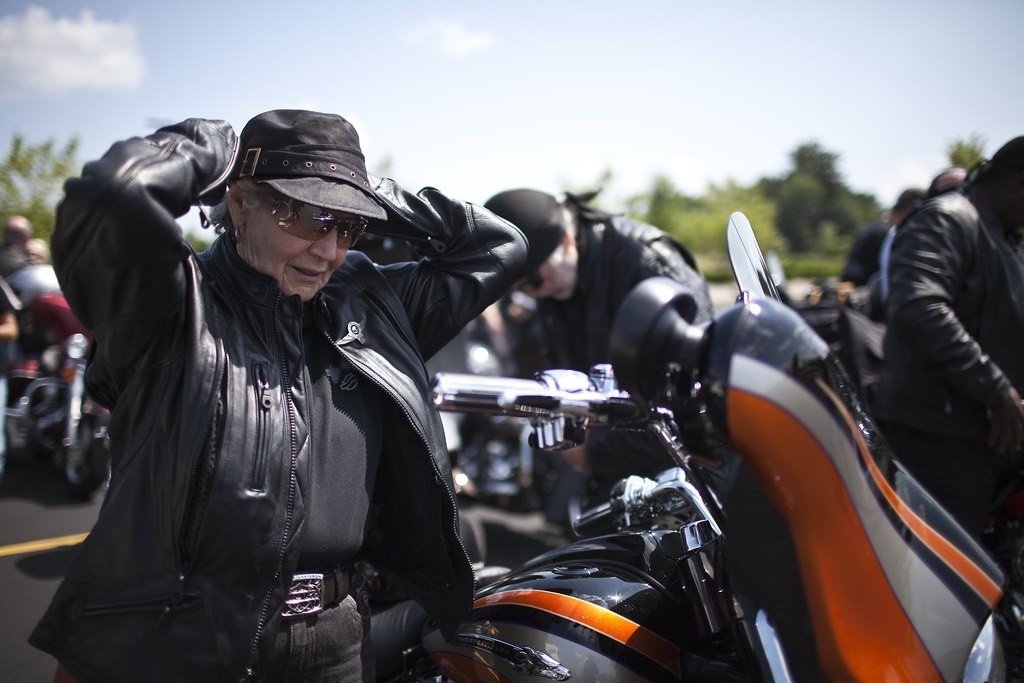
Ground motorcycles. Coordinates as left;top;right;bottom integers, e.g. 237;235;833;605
0;204;1023;683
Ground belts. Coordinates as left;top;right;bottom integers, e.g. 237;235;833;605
283;568;351;621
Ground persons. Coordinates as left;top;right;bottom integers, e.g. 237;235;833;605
838;135;1024;577
28;110;528;683
471;189;712;545
0;215;50;500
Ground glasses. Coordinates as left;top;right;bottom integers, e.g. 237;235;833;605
241;186;366;248
521;271;543;292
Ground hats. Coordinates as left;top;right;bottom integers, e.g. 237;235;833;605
893;189;925;209
482;189;565;278
234;109;387;220
